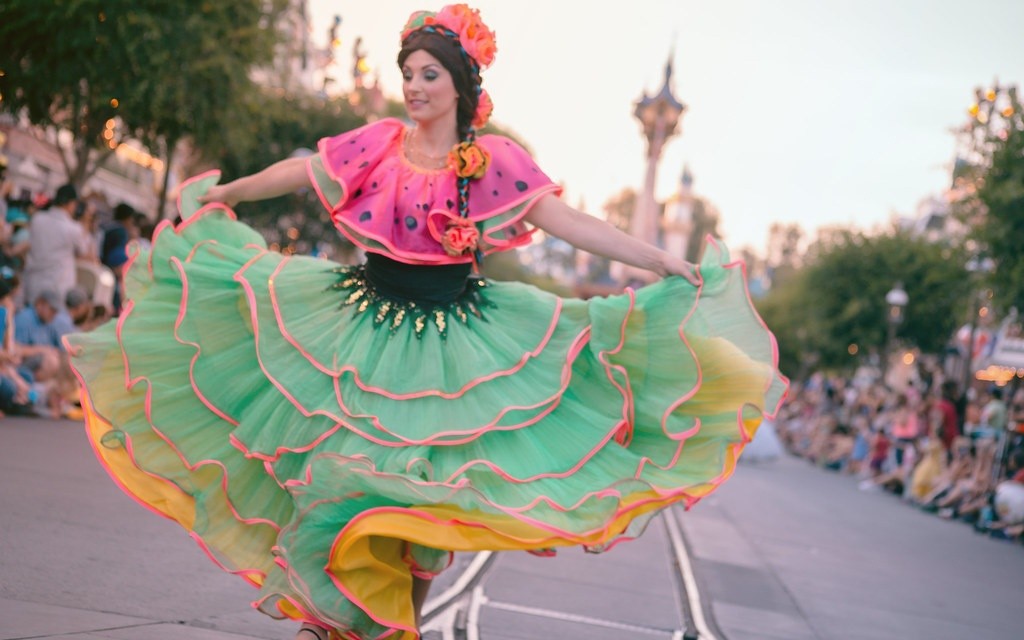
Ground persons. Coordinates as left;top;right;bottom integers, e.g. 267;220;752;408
195;1;705;640
772;350;1024;542
0;163;181;423
